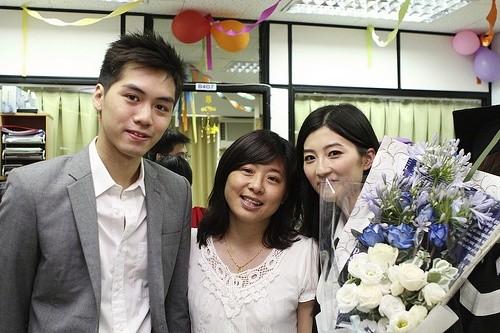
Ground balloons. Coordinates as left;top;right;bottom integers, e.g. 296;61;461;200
472;47;500;82
453;30;480;56
210;19;249;52
171;10;210;44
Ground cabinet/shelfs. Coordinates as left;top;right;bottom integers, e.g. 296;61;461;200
0;113;53;180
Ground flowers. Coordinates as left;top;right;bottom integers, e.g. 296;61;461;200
316;134;496;333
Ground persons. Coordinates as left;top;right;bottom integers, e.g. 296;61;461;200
154;153;208;228
187;128;320;333
0;22;193;333
293;102;380;333
146;127;192;164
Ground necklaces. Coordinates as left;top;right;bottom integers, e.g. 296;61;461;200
222;235;264;274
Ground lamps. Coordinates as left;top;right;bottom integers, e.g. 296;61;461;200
201;96;219;143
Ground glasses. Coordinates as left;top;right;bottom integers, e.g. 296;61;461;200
161;153;191;162
